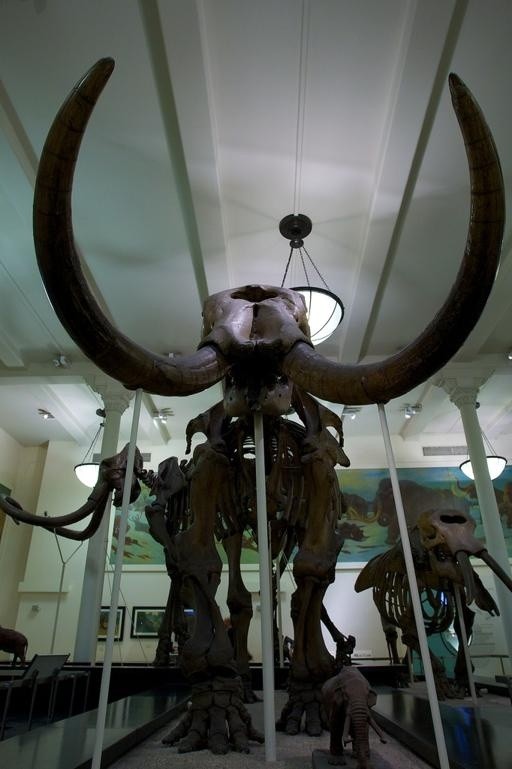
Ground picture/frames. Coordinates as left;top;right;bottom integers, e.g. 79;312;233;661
84;604;200;641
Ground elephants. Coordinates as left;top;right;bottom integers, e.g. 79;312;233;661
320;667;388;769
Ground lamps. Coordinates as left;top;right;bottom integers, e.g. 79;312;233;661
263;208;343;349
72;408;133;493
455;399;507;483
